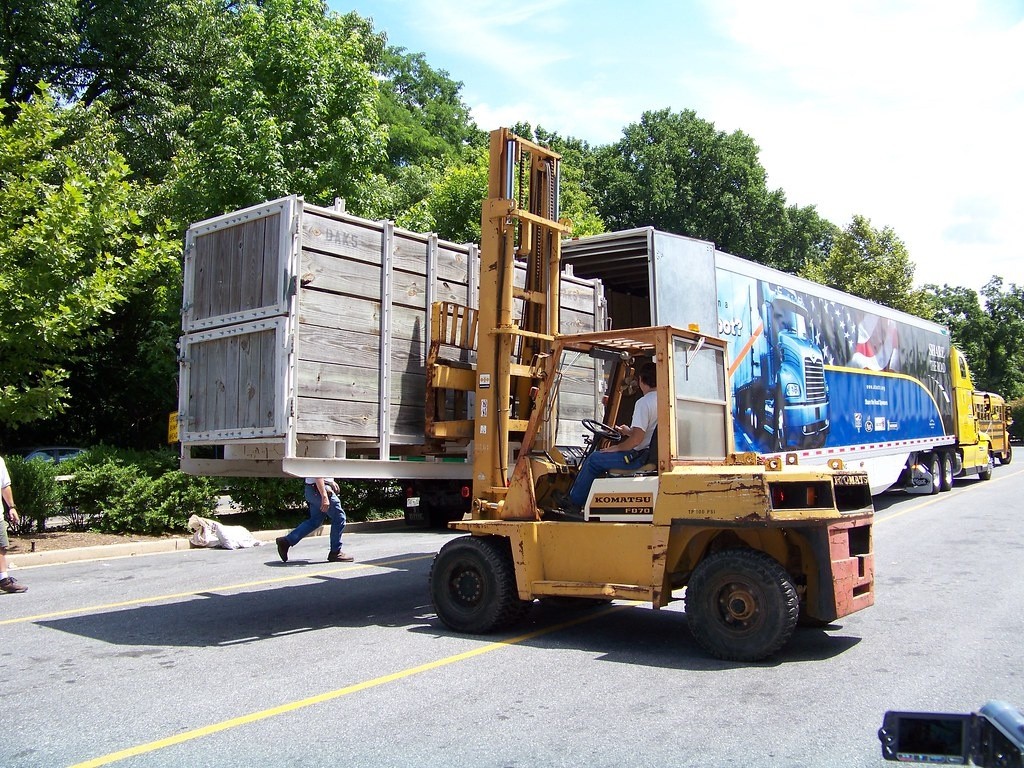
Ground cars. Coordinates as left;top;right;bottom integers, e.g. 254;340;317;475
23;447;88;466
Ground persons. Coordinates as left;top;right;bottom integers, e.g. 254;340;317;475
553;361;658;514
0;457;28;593
276;478;354;562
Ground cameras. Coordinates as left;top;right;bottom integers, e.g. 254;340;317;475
877;701;1024;768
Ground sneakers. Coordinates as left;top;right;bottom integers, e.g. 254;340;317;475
0;576;28;593
276;537;291;563
328;551;355;562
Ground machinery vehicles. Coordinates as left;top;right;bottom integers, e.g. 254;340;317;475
394;133;876;658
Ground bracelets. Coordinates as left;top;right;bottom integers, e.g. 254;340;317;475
8;505;16;510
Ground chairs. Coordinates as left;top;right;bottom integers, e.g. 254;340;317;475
607;423;659;477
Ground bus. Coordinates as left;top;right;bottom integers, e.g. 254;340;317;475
973;390;1012;464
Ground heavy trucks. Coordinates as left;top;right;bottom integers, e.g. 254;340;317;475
510;226;991;500
733;285;830;452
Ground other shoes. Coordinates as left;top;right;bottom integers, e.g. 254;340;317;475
551;488;582;515
